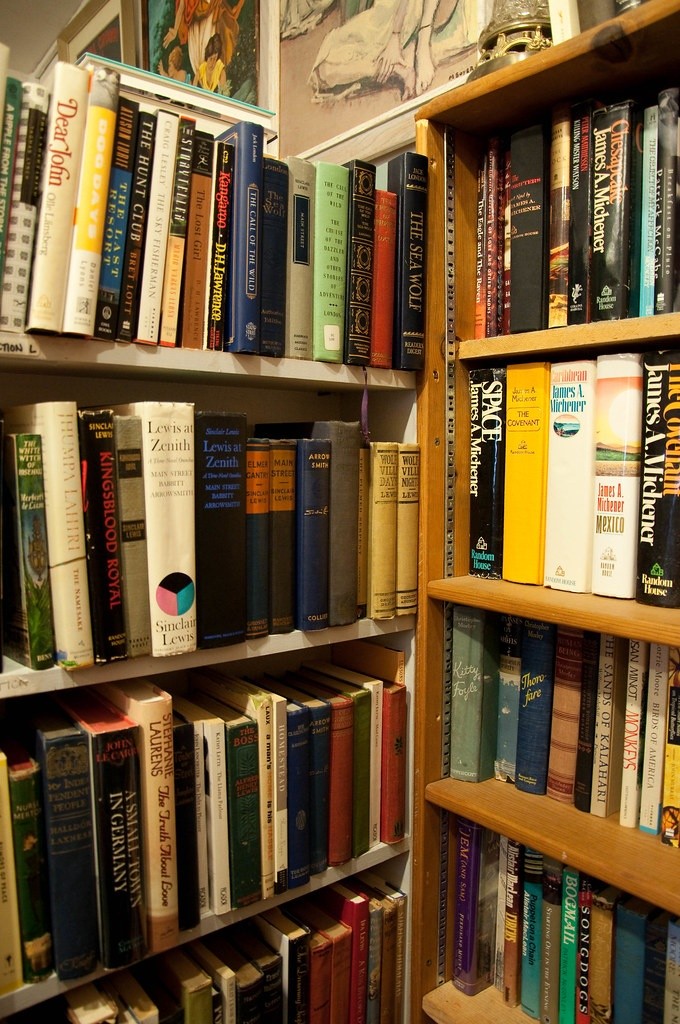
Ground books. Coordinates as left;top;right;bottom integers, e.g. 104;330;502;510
68;52;277;143
0;66;680;1024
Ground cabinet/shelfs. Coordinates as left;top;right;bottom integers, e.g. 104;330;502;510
408;0;680;1024
0;334;416;1024
